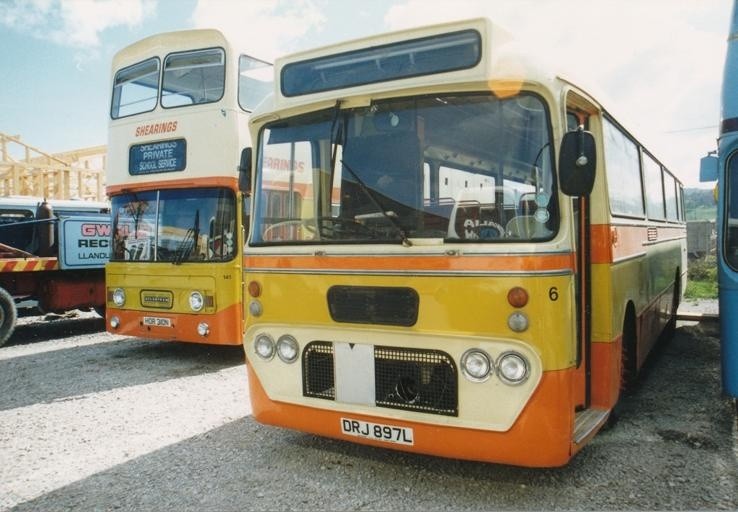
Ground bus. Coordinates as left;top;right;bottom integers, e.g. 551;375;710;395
101;24;303;347
243;16;687;470
696;0;737;399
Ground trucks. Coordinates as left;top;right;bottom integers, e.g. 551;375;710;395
0;195;111;349
